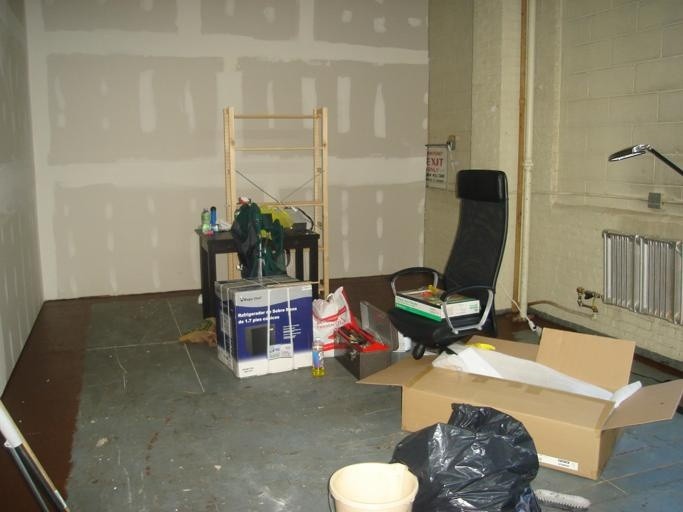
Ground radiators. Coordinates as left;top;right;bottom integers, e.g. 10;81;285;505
601;229;683;327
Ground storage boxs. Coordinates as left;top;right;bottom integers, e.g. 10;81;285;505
214;274;314;380
333;301;395;380
356;327;683;480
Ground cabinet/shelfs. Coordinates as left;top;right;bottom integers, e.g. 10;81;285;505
223;105;329;298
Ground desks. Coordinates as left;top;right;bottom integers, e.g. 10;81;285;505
194;227;319;321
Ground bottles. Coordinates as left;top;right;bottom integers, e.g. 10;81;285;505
201;208;211;229
210;206;216;224
311;337;326;378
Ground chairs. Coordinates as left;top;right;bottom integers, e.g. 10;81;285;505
388;170;509;359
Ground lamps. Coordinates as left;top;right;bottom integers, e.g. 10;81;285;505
609;144;683;176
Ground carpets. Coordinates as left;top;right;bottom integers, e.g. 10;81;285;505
65;298;683;511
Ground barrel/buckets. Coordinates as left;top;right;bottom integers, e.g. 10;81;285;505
327;461;419;512
